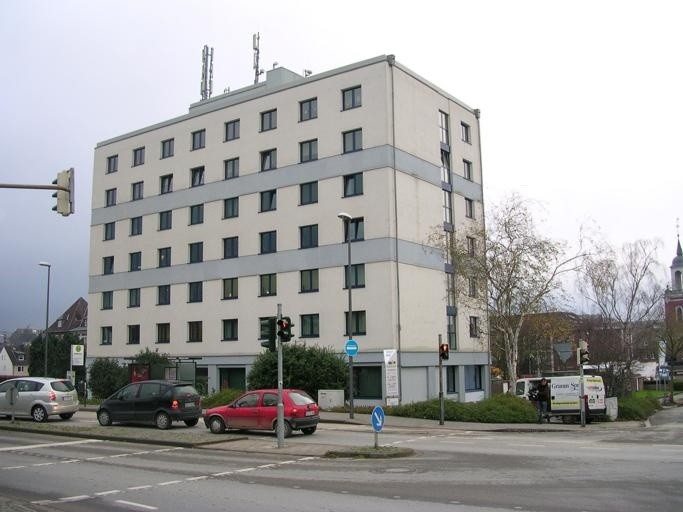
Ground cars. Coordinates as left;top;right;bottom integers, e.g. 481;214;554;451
94;378;202;430
202;387;319;438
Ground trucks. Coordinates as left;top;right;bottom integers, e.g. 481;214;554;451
507;374;605;423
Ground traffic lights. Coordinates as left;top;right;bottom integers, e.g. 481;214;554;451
441;343;449;361
579;348;588;366
277;317;292;343
259;318;276;352
51;169;71;215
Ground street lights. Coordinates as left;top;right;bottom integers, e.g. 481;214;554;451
36;261;51;378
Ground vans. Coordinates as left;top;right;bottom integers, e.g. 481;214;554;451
0;377;79;422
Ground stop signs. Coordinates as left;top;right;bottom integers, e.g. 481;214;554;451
345;339;359;357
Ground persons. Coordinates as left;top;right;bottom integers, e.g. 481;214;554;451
535;378;550;424
